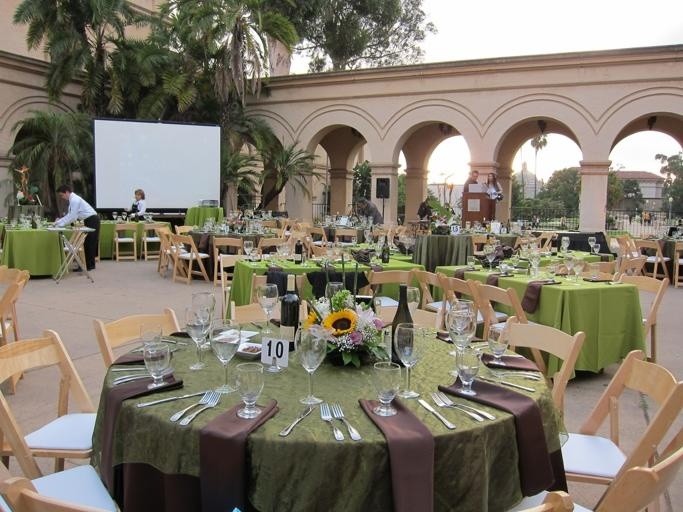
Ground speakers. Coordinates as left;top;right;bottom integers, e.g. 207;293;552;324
376;178;390;198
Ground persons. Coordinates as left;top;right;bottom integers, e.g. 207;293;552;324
484;172;503;201
560;215;567;228
47;185;101;272
628;210;652;227
676;220;683;236
519;212;542;230
355;197;385;226
416;197;432;220
123;188;147;221
462;169;478;192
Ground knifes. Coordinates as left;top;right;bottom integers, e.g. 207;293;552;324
417;399;456;430
278;405;315;438
136;392;207;409
479;376;535;393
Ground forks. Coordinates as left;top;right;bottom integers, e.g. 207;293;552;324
179;392;221;426
488;369;541;381
437;392;495;421
331;402;361;441
430;393;483;423
169;390;213;422
319;403;344;441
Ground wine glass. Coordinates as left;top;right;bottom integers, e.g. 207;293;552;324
394;323;421;399
210;318;240;394
545;264;556;283
235;362;264;420
255;282;278;335
592;243;600;255
121;212;127;223
511;255;519;271
454;347;479;397
185;306;210;371
243;240;252;263
571;260;584;286
372;361;400;418
561;237;569;255
466;255;475;270
486;323;509;366
446;299;476;363
483;247;495;273
269;252;277;268
141;323;162;349
519;240;528;257
562;257;573;280
192;293;215;351
325;281;344;298
588;236;595;253
112;212;117;223
351;231;381;258
142;344;170;390
8;213;42;229
528;254;539;279
498;260;508;277
294;324;327;405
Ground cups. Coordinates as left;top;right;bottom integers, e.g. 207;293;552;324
323;212;373;227
143;211;153;223
203;209;271;234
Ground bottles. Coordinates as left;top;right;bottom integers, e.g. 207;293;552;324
134;210;138;222
381;235;388;263
279;275;300;352
294;239;301;264
550;234;558;255
390;285;414;368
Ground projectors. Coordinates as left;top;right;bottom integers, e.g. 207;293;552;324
199;199;218;207
